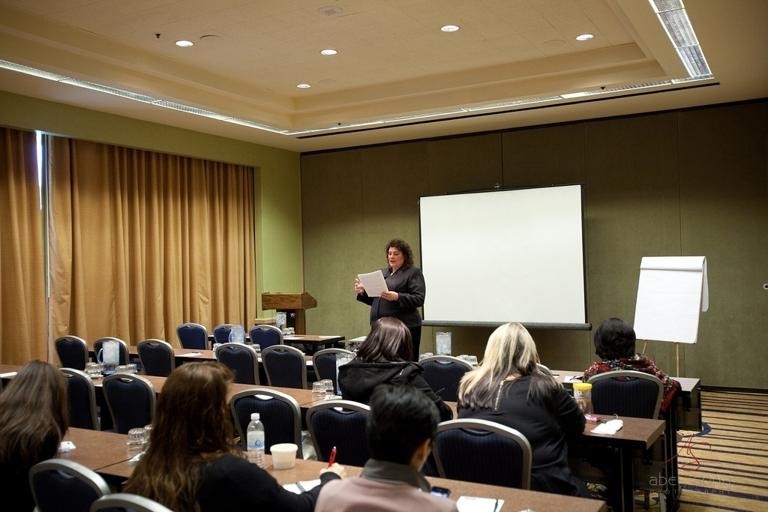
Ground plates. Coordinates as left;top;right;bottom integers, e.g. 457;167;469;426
89;374;103;378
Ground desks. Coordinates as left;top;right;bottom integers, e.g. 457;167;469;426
550;370;703;436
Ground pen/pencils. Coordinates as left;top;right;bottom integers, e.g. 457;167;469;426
493;499;498;512
327;446;336;468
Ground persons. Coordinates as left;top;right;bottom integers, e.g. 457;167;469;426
122;357;346;512
455;320;608;501
352;239;426;366
1;358;68;511
337;316;453;426
314;381;458;512
583;317;681;425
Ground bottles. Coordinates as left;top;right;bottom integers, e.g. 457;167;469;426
246;413;266;470
572;382;592;414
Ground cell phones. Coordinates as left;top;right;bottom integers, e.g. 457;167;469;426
430;486;450;498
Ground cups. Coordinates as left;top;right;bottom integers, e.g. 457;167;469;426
268;443;298;470
126;423;154;459
456;355;477;365
116;363;138;375
434;331;451;364
311;378;334;401
85;362;103;374
97;340;121;368
282;327;295;336
253;344;261;357
228;325;245;345
334;352;355;396
275;312;287;330
